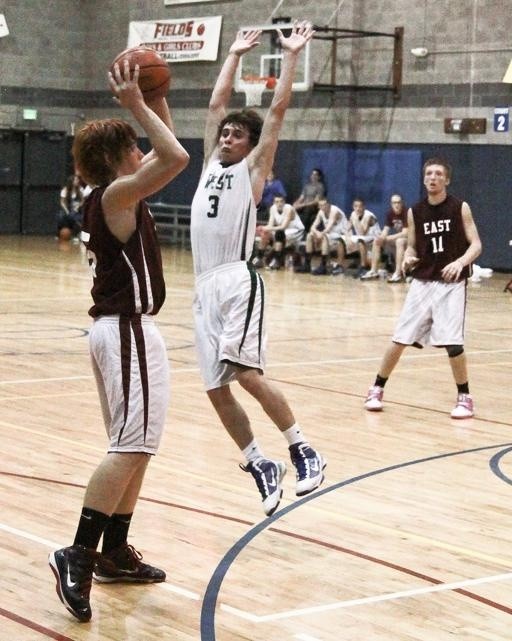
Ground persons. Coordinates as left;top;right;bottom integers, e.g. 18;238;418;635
192;18;328;518
252;168;408;283
364;158;482;418
82;185;99;198
48;60;191;623
53;175;85;241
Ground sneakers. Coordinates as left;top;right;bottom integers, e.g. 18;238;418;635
287;442;327;496
251;257;264;268
92;544;167;582
352;265;379;280
295;266;345;274
270;260;280;268
389;273;404;282
365;386;383;410
47;544;97;622
239;457;286;516
451;394;475;419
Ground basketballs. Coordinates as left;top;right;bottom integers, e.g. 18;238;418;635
60;227;70;239
106;49;174;106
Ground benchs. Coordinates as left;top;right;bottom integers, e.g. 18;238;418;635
142;201;386;263
